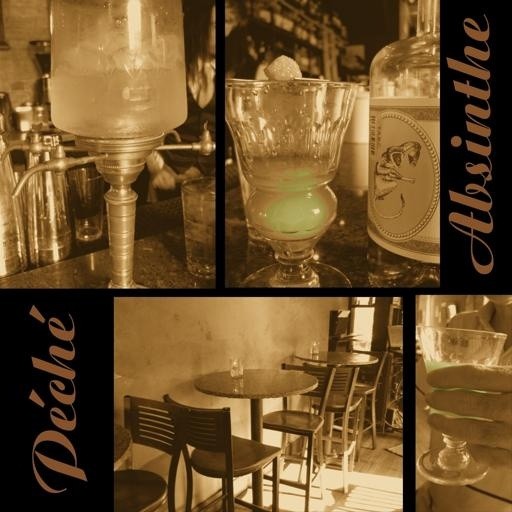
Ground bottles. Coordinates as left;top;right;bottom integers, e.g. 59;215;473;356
366;0;441;288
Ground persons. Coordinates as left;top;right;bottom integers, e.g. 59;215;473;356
416;295;512;512
146;0;233;201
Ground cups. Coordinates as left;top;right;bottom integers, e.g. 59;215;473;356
0;133;72;277
310;341;320;356
181;176;216;279
229;359;244;379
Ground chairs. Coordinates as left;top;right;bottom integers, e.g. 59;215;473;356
112;350;387;512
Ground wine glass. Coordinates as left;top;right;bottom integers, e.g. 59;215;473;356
417;326;508;487
51;1;187;289
226;80;357;288
